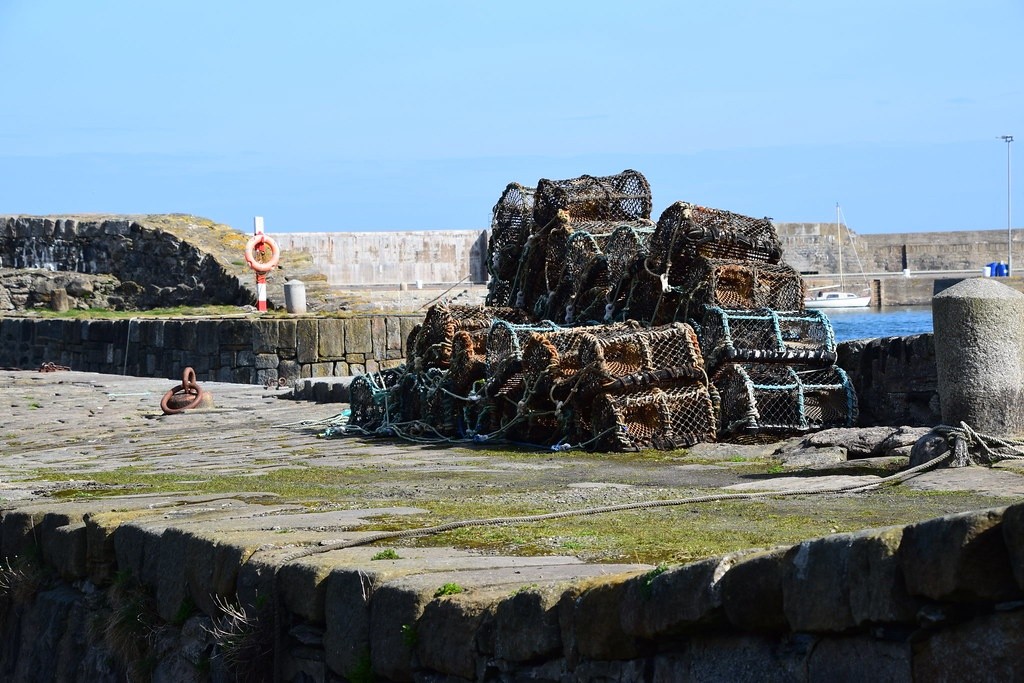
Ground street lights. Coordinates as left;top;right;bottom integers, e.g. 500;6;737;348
1000;133;1014;277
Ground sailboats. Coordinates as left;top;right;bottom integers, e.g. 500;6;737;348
803;202;874;308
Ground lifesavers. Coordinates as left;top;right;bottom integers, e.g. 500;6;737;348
245;234;280;272
